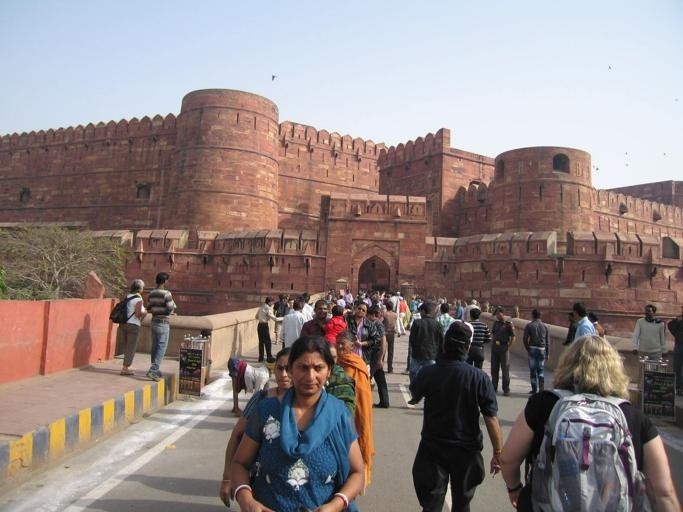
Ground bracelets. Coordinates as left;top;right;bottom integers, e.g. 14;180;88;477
495;449;502;453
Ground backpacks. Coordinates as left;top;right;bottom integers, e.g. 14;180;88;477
110;295;140;324
529;389;651;512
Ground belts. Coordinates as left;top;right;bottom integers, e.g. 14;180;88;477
471;345;483;350
493;340;507;345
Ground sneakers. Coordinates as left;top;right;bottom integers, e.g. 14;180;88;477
120;369;136;376
404;369;409;375
504;390;510;396
373;402;389;408
530;390;537;394
147;371;162;381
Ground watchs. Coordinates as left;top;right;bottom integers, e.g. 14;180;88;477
508;483;523;493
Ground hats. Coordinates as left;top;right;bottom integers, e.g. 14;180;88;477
493;309;504;315
449;321;473;345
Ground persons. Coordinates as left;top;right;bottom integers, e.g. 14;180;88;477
562;302;606;346
407;321;504;512
400;293;516;396
522;309;550;394
220;331;375;512
257;287;407;408
117;278;148;375
632;304;683;395
145;271;175;382
499;335;683;512
